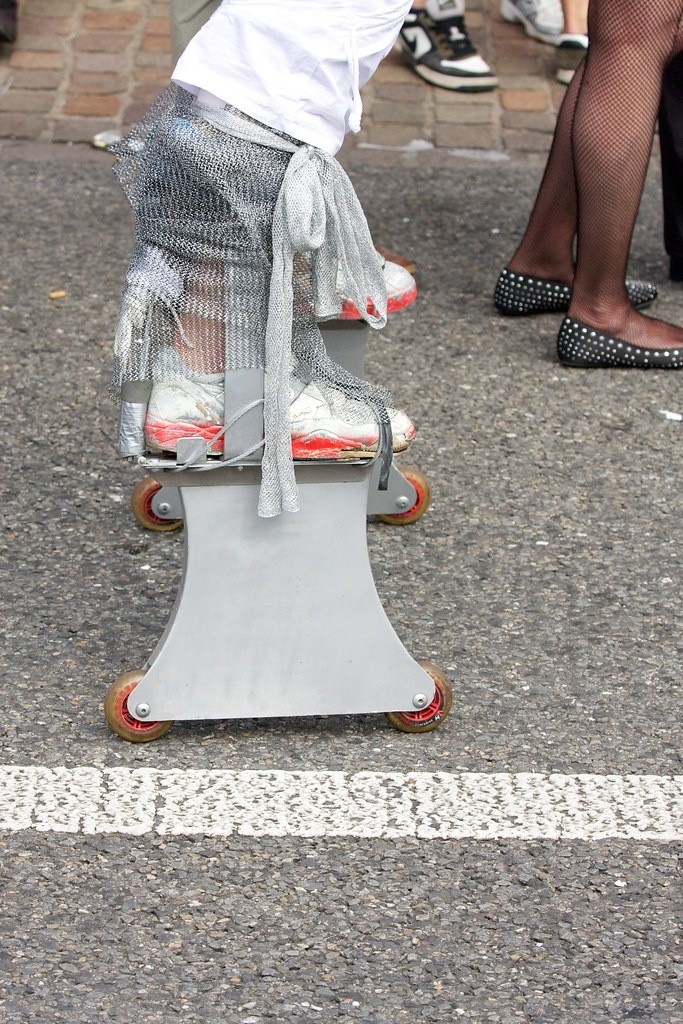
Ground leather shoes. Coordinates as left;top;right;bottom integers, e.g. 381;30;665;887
493;265;657;318
558;317;683;371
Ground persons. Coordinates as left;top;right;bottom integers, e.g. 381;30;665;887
658;52;683;285
397;1;590;94
494;1;683;371
136;0;418;459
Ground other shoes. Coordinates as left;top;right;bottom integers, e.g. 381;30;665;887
375;246;417;275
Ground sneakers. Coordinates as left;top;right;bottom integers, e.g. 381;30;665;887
501;0;565;47
393;0;501;96
333;253;420;322
554;34;591;86
138;345;415;463
